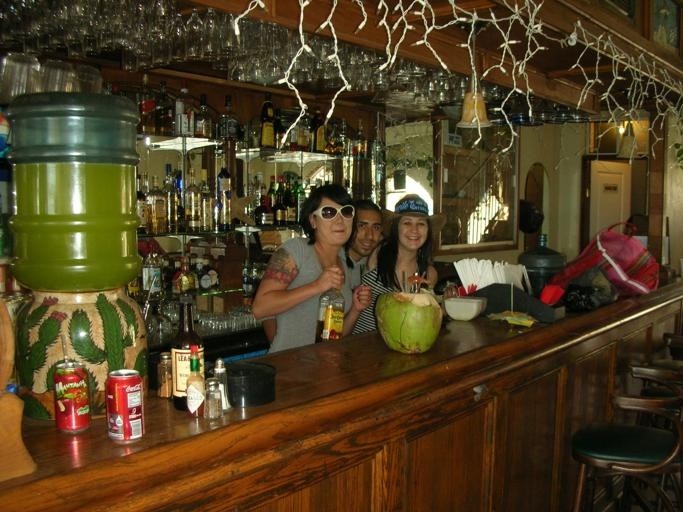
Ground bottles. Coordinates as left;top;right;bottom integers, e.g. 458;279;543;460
212;357;231;411
102;69;387;303
170;301;204;412
155;351;172;399
202;377;223;421
185;345;204;419
145;304;172;349
312;265;346;345
0;153;26;299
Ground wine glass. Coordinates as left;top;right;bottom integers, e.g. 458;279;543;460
0;0;586;129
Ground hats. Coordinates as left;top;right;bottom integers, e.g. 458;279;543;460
394;193;430;219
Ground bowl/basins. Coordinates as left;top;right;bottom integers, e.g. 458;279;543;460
206;362;277;409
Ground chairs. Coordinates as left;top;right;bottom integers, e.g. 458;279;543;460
612;368;682;512
567;403;682;511
634;329;683;427
625;328;682;436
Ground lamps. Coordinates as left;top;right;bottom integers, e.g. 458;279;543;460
457;10;494;131
618;66;642;160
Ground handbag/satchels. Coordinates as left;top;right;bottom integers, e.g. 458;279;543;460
549;220;661;298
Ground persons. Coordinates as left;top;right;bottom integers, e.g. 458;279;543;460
351;193;439;338
251;182;370;353
622;210;649;254
260;200;383;342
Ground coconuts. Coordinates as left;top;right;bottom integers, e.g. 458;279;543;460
375;291;445;354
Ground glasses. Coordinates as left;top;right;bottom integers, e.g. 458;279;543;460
307;204;356;222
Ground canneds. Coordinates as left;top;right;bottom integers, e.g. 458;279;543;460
105;370;146;445
53;362;91;434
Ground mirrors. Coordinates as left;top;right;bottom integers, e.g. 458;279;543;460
434;109;524;257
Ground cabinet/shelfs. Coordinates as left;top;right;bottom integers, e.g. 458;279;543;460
0;48;387;327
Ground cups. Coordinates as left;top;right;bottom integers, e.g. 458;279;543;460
198;306;261;335
